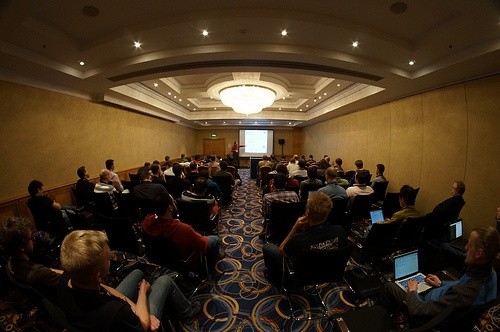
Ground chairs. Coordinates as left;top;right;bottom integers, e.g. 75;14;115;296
258;166;500;332
0;155;238;332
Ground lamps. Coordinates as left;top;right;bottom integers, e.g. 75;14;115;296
220;86;277;114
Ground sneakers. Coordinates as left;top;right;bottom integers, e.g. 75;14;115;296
185;299;202;325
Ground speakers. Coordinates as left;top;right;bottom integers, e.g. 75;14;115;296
278;139;285;145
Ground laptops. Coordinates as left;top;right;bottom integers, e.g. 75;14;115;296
448;218;468;250
393;249;434;296
369;209;385;225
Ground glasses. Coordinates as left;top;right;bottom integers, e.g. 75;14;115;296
26;235;36;244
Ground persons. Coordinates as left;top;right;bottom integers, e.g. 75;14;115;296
427;203;500;271
256;152;389;222
429;180;466;224
101;157;124;192
360;185;423;250
231;142;241;167
132;167;180;212
142;190;227;270
76;166;95;192
0;216;72;295
47;227;202;332
182;178;222;225
93;169;123;211
141;151;242;199
378;224;498;332
262;192;343;286
27;180;90;229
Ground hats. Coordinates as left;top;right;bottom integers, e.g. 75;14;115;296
132;183;169;208
